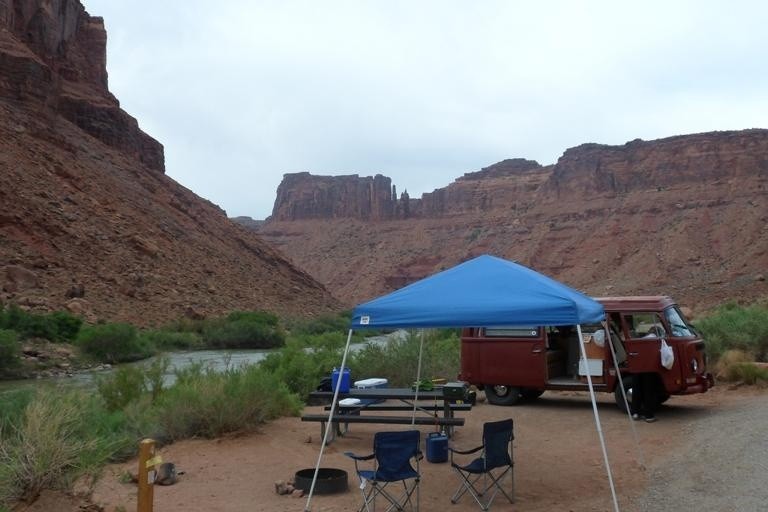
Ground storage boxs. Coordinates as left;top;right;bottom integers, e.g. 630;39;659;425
354;377;389;405
339;398;361;417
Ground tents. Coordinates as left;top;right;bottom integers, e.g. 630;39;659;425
301;250;631;511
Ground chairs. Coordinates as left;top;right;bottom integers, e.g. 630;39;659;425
344;429;420;511
443;418;515;512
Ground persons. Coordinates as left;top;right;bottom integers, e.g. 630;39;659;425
630;326;678;418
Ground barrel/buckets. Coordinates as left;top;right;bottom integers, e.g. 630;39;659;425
425;431;449;464
330;366;351;394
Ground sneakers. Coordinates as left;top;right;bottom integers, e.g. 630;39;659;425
645;416;657;423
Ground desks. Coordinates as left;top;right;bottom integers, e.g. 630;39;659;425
308;388;471;438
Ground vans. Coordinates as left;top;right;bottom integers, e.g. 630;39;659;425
457;295;714;413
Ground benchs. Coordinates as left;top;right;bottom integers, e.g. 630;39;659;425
324;403;472;432
301;414;465;446
